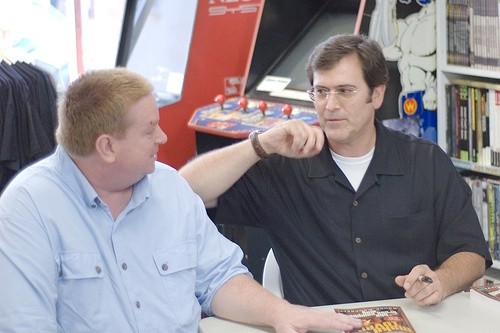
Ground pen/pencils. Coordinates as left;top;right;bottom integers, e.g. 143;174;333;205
418;275;433;283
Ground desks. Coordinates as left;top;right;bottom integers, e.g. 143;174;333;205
198;292;500;333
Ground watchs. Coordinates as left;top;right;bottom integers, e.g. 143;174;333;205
248;129;269;160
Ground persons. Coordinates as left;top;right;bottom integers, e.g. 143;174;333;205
0;69;362;333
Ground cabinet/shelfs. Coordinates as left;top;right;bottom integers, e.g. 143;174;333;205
435;0;500;270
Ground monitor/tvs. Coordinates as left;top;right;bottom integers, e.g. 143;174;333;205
243;0;365;106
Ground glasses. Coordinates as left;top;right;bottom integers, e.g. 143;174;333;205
306;85;364;103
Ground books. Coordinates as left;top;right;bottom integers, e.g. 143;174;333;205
458;170;500;261
446;0;500;73
446;83;500;168
471;273;500;287
177;34;493;306
